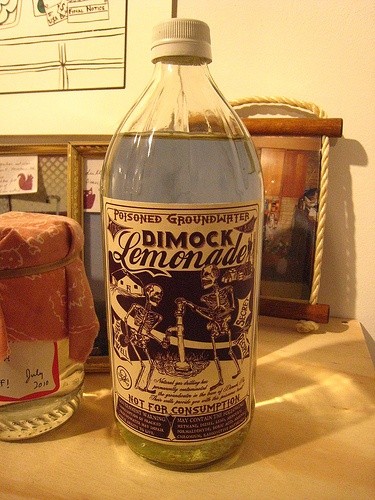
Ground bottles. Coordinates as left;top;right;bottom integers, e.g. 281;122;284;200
94;16;265;471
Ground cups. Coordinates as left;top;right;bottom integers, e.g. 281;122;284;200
0;209;96;442
